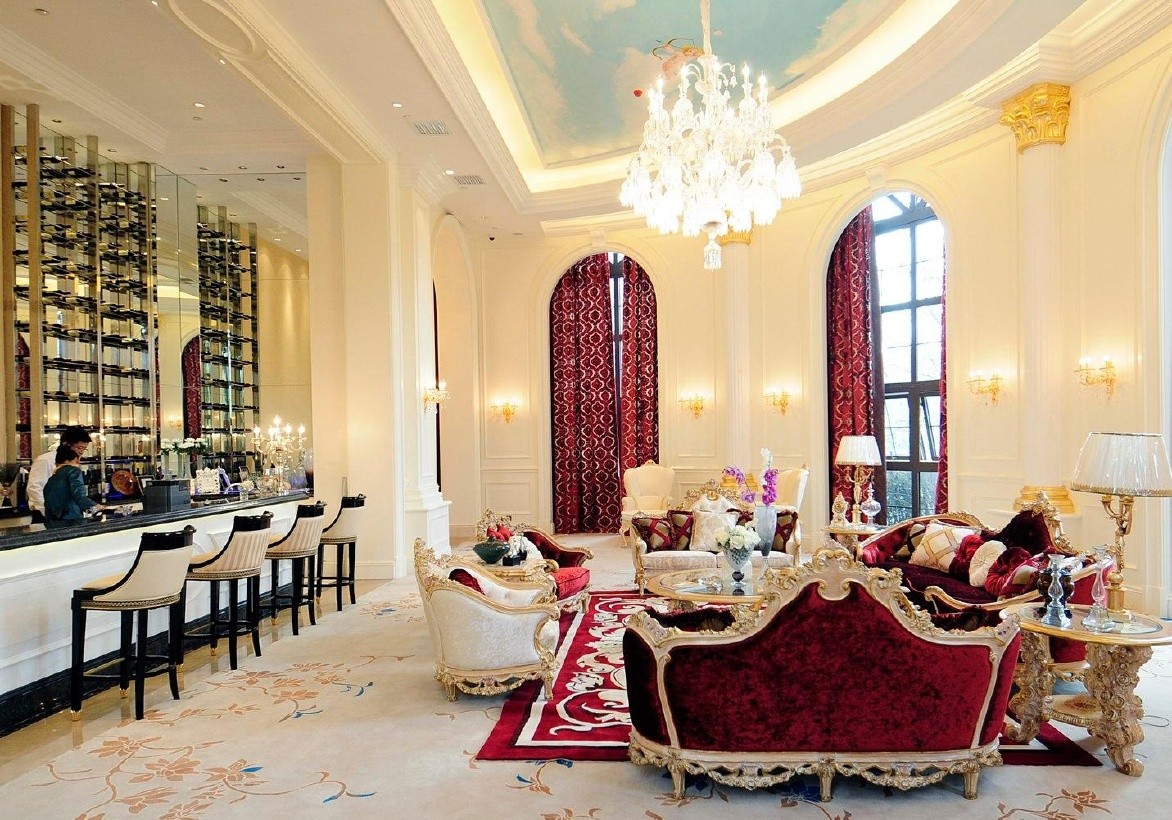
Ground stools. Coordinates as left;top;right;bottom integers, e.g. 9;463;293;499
165;509;271;673
297;488;365;613
69;526;197;731
259;498;324;638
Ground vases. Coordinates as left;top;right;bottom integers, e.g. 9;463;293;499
754;507;779;579
722;544;754;586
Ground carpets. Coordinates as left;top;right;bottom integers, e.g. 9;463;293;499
472;589;1105;771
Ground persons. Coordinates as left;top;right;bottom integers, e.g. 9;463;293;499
25;426;93;524
43;445;105;529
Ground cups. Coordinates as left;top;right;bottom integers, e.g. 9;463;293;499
239;486;249;501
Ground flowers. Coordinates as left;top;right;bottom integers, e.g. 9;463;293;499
723;447;780;506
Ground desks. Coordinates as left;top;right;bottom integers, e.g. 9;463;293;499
998;601;1172;779
821;525;892;556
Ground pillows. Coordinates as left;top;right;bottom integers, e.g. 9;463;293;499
892;518;1083;599
928;605;991;632
632;509;799;554
647;606;737;635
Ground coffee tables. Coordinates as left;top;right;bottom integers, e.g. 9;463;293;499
647;567;789;610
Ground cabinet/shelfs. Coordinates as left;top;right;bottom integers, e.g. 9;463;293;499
3;105;261;495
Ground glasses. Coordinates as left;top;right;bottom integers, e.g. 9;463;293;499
69;443;88;452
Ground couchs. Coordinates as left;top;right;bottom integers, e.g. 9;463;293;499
411;536;565;703
623;483;802;593
850;491;1115;692
619;544;1023;804
478;508;592;621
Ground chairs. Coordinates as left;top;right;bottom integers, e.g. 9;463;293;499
618;460;675;548
757;467;808;557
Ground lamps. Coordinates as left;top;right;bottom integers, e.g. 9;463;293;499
1070;430;1172;622
966;346;1126;404
488;388;524;420
678;377;798;419
617;2;802;264
835;434;882;525
423;376;449;415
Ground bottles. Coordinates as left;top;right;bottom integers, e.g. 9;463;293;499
198;220;263;484
258;465;286;496
14;138;158;497
